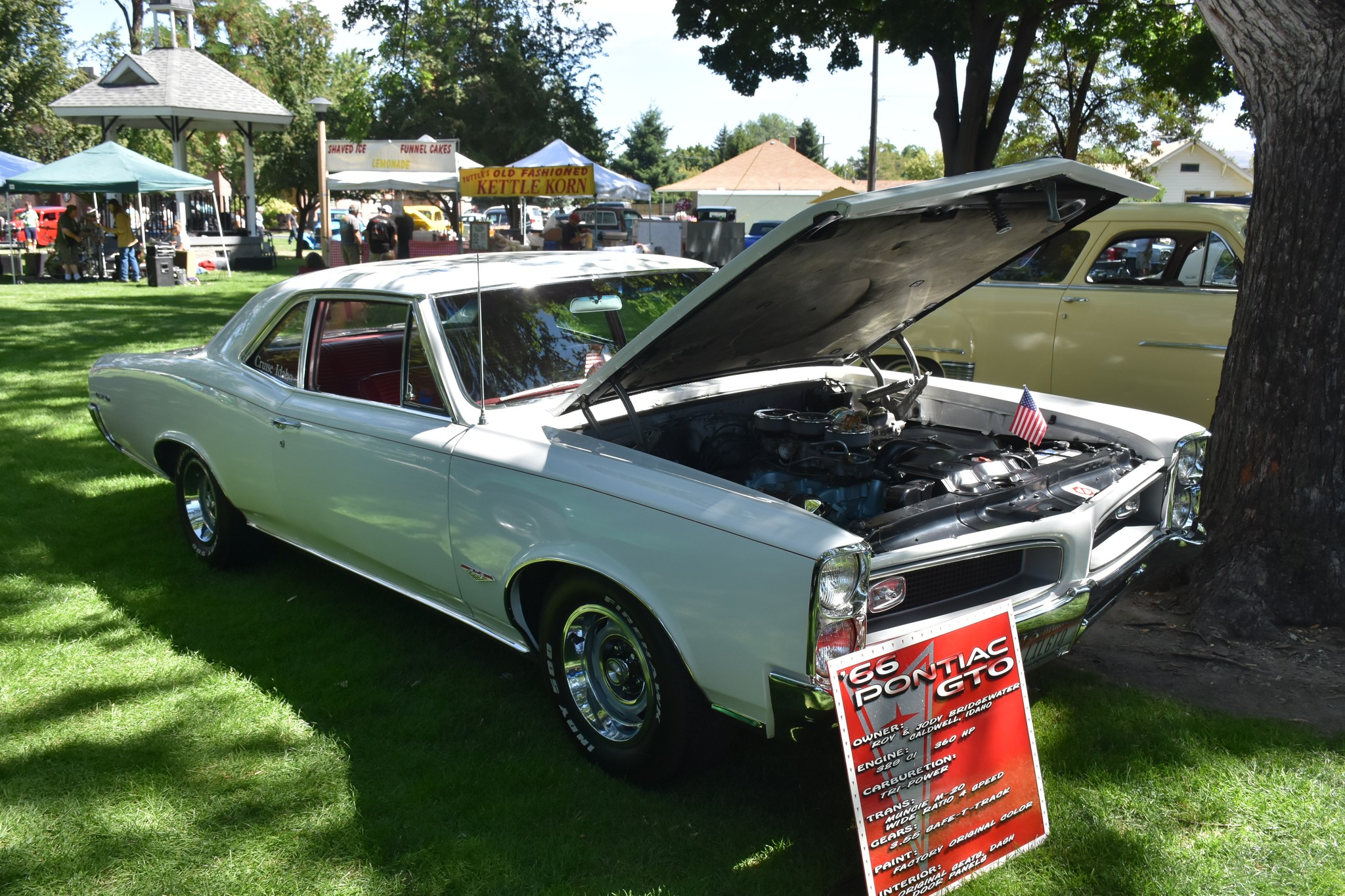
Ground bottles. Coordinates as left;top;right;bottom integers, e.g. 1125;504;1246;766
432;230;449;242
204;220;208;232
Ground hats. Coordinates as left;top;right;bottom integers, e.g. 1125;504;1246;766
382;205;392;213
569;213;581;223
292;207;299;212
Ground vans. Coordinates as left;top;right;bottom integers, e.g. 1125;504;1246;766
586;202;632;210
482;204;544;232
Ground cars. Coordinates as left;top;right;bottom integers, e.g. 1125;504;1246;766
303;209;367;249
87;160;1214;785
3;206;68;247
402;204;463;235
147;199;236;230
546;207;664;246
460;213;493;234
845;187;1254;432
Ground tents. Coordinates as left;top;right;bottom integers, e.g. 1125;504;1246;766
505;138;652;251
0;140;231;285
326;135;484;269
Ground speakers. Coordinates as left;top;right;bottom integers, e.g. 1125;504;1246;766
25;253;49;274
146;256;176;287
0;254;23;275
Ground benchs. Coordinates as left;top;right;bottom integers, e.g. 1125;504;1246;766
263;326;481;399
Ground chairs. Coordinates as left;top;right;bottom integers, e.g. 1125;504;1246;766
359;366;443;414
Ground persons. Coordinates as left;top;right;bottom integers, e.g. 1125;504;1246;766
171;219;191;252
235;208;246;230
288;207;300;244
255;206;265;236
562;213;593;251
339;204;362;266
142;206;150;232
395;214;414;259
83;207;96;225
20;202;40;253
129;202;141;235
53;205;82;282
163;206;174;233
103;199;140;283
365;204;398;262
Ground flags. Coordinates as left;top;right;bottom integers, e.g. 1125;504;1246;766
1009;386;1047;446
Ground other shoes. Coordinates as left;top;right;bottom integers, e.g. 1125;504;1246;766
64;278;87;283
